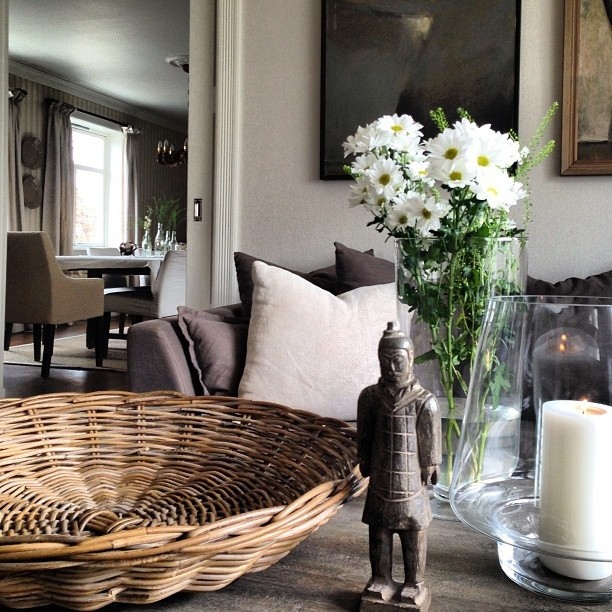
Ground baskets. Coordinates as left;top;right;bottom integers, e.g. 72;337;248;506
1;390;371;607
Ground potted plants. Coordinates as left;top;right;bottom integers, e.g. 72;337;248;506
169;202;185;250
163;211;170;250
152;193;179;251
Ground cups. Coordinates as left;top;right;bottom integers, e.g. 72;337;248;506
449;291;612;601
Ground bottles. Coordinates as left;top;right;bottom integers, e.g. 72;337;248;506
142;230;151;250
170;231;179;250
154;222;164;255
164;231;170;252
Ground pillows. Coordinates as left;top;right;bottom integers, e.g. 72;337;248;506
528;268;612;409
235;258;419;422
333;241;399;295
178;306;252;398
230;249;361;314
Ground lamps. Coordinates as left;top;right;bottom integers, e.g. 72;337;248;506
156;56;189;169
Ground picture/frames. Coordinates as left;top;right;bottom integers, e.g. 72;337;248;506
558;0;612;176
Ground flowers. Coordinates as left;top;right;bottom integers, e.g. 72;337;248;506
142;207;153;230
334;99;560;240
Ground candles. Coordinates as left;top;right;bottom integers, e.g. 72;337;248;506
537;398;612;555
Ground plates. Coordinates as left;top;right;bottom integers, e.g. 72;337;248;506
20;137;45;168
22;173;43;209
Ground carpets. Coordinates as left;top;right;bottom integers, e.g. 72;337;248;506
4;324;128;378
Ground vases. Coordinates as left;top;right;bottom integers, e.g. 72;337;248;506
142;229;152;256
396;239;529;520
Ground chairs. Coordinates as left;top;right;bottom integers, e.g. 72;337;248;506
8;231;106;367
104;250;184;358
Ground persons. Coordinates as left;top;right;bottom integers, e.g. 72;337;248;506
356;320;442;608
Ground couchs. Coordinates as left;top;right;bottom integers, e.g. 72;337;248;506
129;244;611;400
